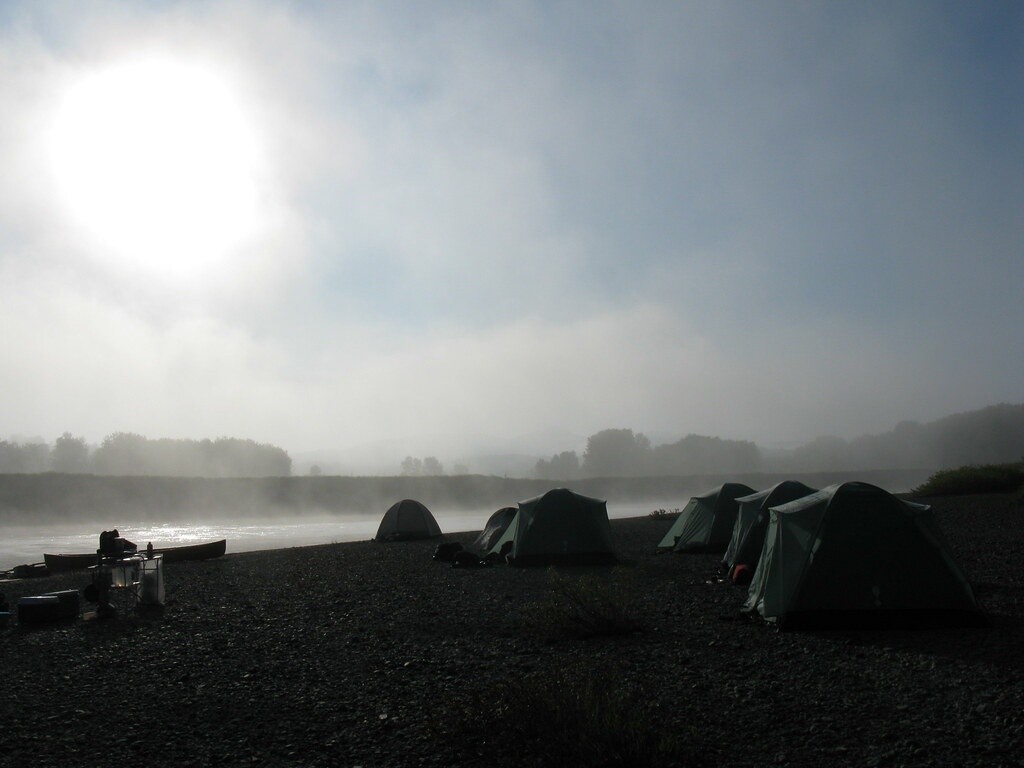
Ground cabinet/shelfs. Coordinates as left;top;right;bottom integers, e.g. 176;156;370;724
97;549;145;621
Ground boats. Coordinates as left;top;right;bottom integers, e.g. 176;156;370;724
43;528;227;572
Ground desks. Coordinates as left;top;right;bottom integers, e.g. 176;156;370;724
88;554;165;601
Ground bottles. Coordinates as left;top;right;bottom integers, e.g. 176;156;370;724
147;542;153;558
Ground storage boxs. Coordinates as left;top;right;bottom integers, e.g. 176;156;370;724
16;590;80;623
0;612;16;624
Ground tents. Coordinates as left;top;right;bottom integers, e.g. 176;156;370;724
375;500;442;541
474;487;616;564
658;478;979;625
